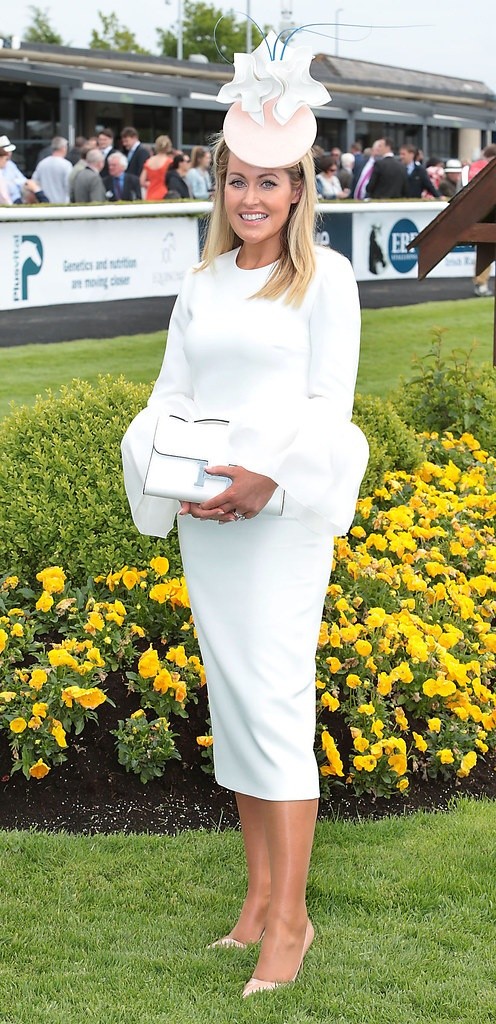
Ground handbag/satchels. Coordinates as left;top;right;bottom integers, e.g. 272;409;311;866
142;417;287;514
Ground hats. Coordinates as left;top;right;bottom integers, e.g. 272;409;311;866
0;135;16;152
444;160;463;172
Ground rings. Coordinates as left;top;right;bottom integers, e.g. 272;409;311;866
232;510;245;521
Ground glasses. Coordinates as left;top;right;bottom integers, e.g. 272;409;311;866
185;159;192;164
330;169;336;172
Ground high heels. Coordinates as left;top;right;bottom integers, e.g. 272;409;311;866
243;918;314;997
208;927;264;950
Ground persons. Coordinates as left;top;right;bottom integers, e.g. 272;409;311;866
0;127;216;206
312;138;496;201
121;131;370;999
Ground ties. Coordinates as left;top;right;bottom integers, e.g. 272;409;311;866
115;178;121;200
356;164;373;200
